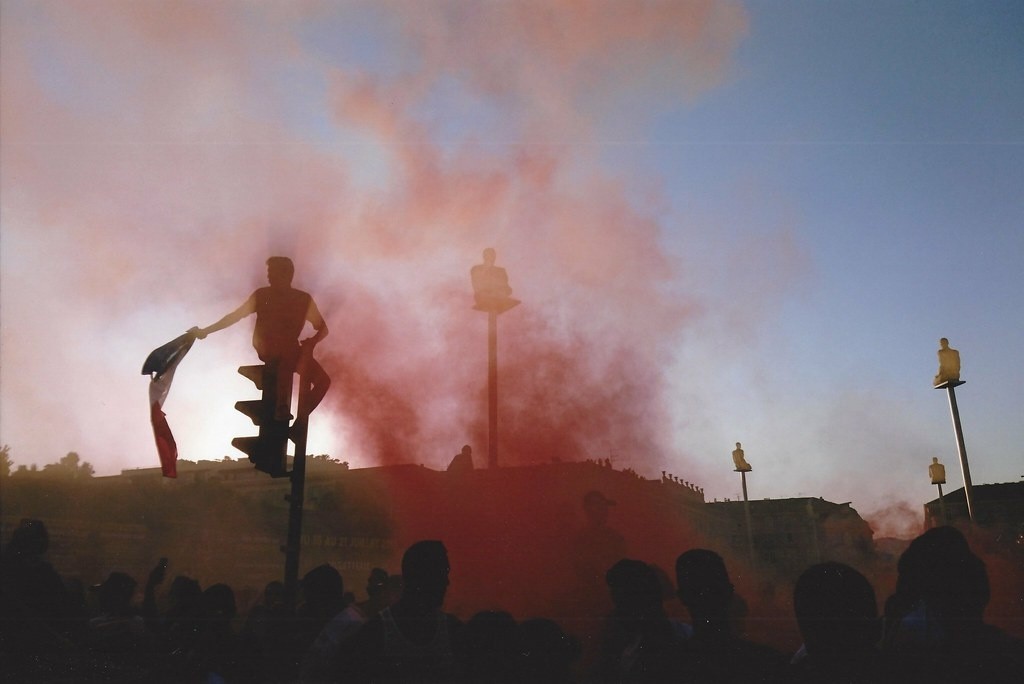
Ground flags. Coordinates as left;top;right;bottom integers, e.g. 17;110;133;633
142;332;201;478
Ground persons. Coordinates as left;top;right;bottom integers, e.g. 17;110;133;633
929;457;946;482
0;519;1024;684
472;249;514;306
190;257;331;445
586;457;612;470
732;443;752;471
934;338;960;387
447;445;473;471
